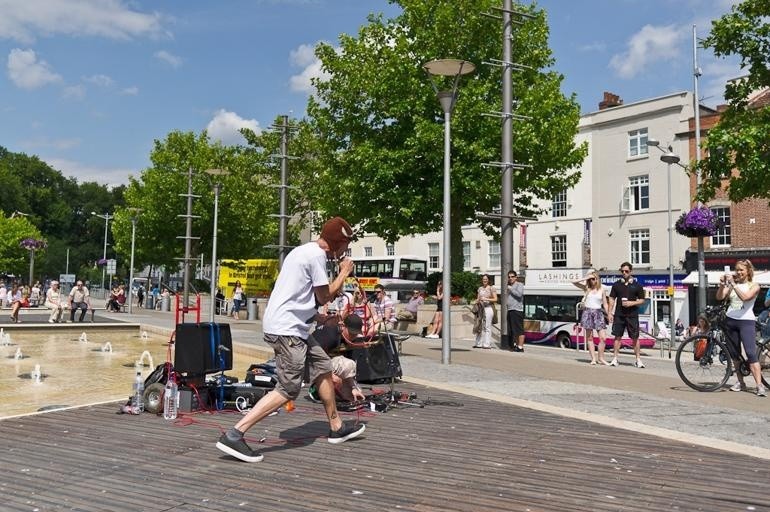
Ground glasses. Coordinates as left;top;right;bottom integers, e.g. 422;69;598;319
375;291;381;293
508;276;516;278
621;270;629;273
588;278;595;281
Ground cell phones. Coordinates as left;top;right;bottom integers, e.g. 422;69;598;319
725;266;730;275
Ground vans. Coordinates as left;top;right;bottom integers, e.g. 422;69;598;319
131;277;149;297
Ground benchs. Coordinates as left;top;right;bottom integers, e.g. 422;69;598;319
1;307;106;323
106;303;128;312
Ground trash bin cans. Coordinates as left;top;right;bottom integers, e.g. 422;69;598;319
247;298;258;321
146;294;153;309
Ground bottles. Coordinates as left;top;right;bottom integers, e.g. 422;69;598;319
285;399;294;411
131;373;145;413
163;376;178;420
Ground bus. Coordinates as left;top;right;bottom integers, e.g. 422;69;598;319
491;284;658;350
318;257;429;316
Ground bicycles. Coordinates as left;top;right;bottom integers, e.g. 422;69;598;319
674;305;769;393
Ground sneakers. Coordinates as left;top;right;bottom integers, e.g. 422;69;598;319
10;317;22;323
730;380;746;391
634;360;645;368
754;383;767;396
591;358;619;366
425;334;439;339
328;423;366;444
215;431;264;462
509;344;524;352
308;381;323;403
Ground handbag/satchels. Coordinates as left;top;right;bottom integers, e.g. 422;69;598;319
244;357;277;387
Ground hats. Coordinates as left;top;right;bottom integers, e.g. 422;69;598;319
344;314;363;334
51;281;59;284
320;217;354;259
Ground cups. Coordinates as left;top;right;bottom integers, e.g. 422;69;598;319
622;298;628;306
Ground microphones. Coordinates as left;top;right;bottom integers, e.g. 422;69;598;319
340;256;358;281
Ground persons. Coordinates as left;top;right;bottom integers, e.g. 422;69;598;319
317;280;444;342
758;308;770;338
675;314;715;361
571;272;613;366
216;278;246;320
472;274;497;348
506;270;525;351
105;282;169;312
608;262;645;368
0;278;53;323
717;259;768;397
216;216;367;463
765;287;770;363
45;281;65;323
68;280;91;323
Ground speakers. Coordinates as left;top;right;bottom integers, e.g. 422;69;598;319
349;335;402;382
174;322;232;383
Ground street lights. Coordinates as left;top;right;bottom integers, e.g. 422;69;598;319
204;167;232;323
421;56;478;363
126;205;146;315
660;153;709;334
91;211;114;290
644;139;676;351
